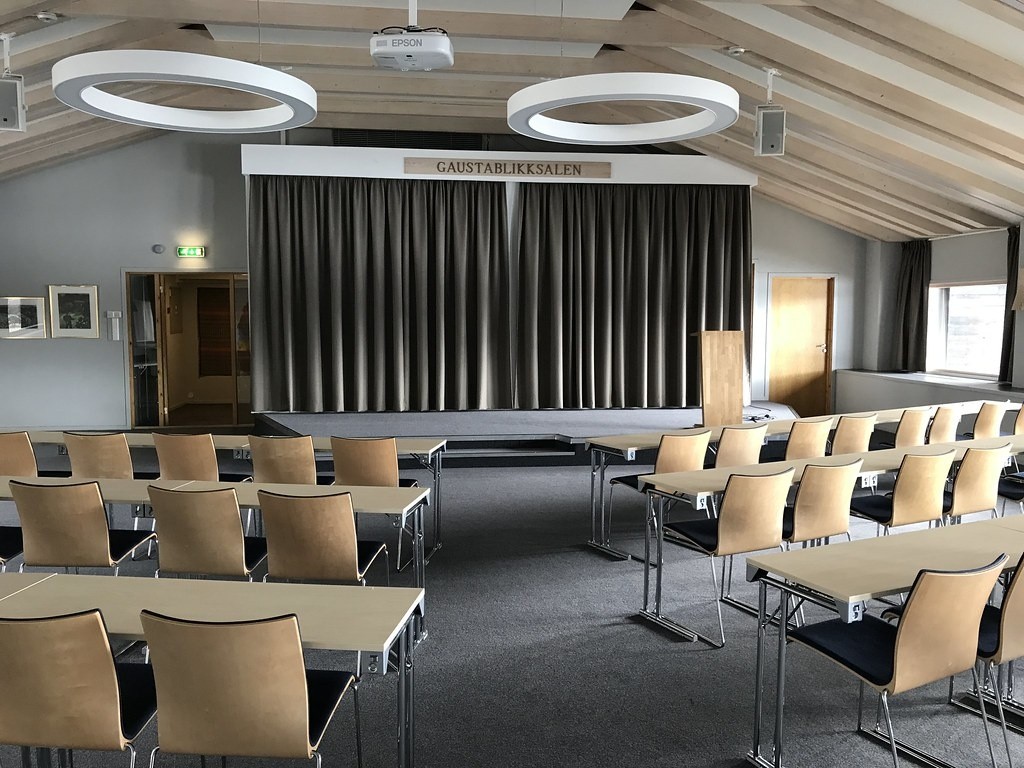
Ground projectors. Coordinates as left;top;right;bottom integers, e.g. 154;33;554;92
370;34;454;72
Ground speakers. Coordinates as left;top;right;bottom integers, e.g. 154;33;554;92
0;76;26;133
755;105;787;156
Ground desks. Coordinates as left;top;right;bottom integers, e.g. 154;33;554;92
585;398;1024;641
745;522;1024;768
2;431;117;454
0;574;426;768
243;435;447;566
167;481;428;660
0;474;196;520
966;514;1024;718
0;572;53;768
124;433;255;461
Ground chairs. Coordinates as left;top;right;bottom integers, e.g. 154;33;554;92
0;429;421;587
140;608;362;768
0;608;205;768
605;400;1024;647
785;553;1009;768
881;553;1024;768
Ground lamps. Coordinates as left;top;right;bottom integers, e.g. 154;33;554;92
508;73;740;146
52;49;318;133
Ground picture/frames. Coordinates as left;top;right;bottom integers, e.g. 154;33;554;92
0;296;46;339
49;285;100;339
